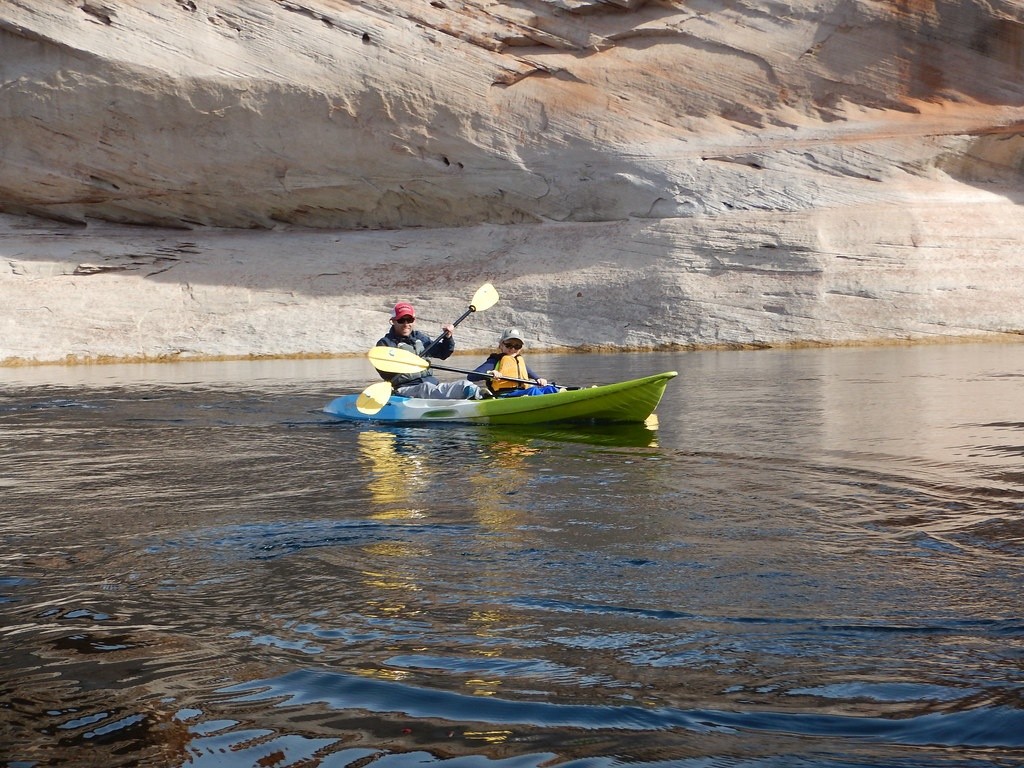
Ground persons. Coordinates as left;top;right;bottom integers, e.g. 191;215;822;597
366;302;483;401
466;327;574;396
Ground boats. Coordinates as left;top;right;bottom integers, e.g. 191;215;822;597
400;412;663;452
313;371;679;425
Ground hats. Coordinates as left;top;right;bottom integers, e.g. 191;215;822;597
499;326;525;346
389;302;415;321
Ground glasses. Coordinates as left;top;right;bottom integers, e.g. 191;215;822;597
502;342;522;350
396;318;415;324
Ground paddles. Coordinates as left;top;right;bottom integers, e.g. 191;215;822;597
367;345;568;387
354;283;502;415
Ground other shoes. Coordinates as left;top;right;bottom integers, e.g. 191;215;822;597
479;388;495;400
558;387;567;393
592;384;598;388
